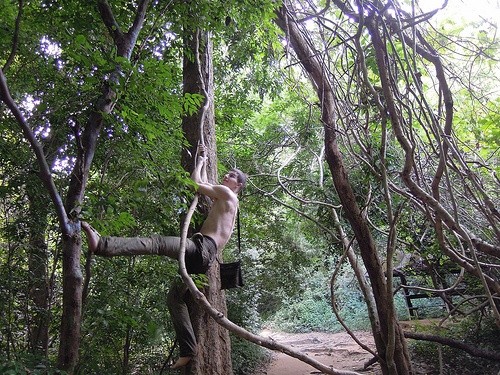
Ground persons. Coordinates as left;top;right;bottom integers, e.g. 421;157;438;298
80;140;247;370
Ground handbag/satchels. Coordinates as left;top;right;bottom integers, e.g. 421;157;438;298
220;260;244;289
